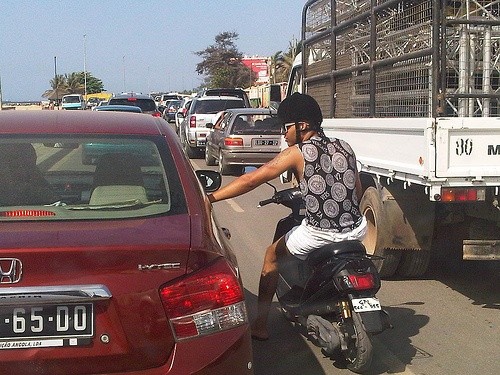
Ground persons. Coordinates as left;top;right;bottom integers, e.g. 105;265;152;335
209;92;368;338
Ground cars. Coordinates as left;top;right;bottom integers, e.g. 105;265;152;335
155;92;193;133
87;97;99;107
205;107;294;183
81;101;161;164
0;107;252;375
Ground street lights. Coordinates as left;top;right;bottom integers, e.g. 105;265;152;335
121;55;126;92
81;34;88;94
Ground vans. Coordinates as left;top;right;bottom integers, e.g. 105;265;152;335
196;87;251;108
106;94;162;116
60;94;86;110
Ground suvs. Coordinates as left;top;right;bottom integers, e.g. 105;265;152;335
174;95;245;159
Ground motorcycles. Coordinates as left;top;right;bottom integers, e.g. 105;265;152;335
241;166;394;374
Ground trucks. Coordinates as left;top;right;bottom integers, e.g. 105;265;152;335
284;0;500;282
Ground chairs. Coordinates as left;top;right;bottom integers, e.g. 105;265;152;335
0;142;59;205
89;153;148;204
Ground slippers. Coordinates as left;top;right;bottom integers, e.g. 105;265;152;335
252;335;269;341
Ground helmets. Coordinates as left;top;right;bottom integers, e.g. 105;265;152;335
277;92;324;123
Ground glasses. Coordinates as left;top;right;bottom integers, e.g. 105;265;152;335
280;121;310;135
